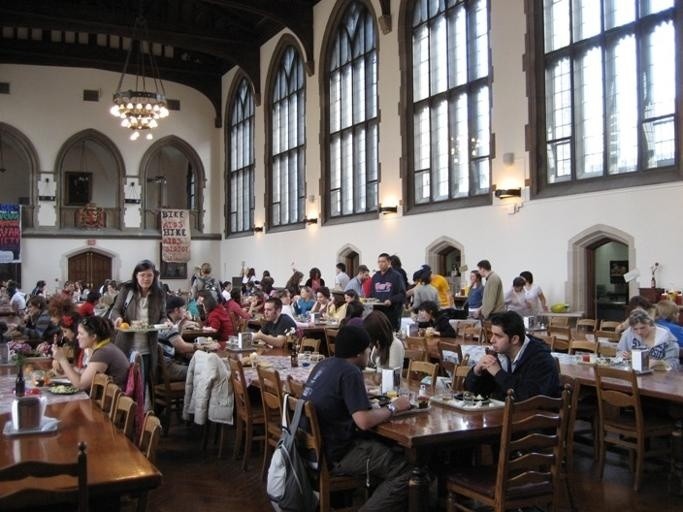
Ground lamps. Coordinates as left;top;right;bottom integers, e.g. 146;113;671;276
378;195;397;215
494;179;522;200
110;0;170;141
304;208;317;225
254;222;263;233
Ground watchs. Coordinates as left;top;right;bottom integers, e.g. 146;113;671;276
386;403;398;416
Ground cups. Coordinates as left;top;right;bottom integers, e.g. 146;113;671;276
301;351;319;367
465;393;474;405
252;359;257;369
194;337;207;343
53;335;63;348
131;319;144;331
574;351;590;362
443;391;454;400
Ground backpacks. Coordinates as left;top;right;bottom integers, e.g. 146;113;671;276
266;394;318;512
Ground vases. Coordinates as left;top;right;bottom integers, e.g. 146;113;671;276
651;272;656;288
14;366;25;397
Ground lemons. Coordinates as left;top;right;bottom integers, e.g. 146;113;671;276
120;322;130;331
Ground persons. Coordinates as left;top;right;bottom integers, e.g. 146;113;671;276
616;297;683;346
462;259;505;321
251;268;335;353
464;311;559;473
160;263;250;381
363;312;405;377
391;254;461;336
50;318;130;408
505;271;551;313
0;275;85;373
616;307;680;367
112;259;173;411
87;274;118;313
292;318;415;511
335;251;406;332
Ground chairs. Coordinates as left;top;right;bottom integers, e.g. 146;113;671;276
147;372;186;434
100;379;123;420
111;391;139;438
0;438;89;512
89;370;115;411
135;410;163;512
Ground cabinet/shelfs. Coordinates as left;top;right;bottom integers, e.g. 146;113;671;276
638;288;664;304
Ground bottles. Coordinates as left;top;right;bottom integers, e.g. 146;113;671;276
399;381;409;401
291;346;298;367
419;385;427;397
16;365;25;397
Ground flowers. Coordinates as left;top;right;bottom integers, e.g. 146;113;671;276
650;262;659;272
5;339;44;369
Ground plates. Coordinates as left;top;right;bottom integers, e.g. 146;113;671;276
47;384;79;395
